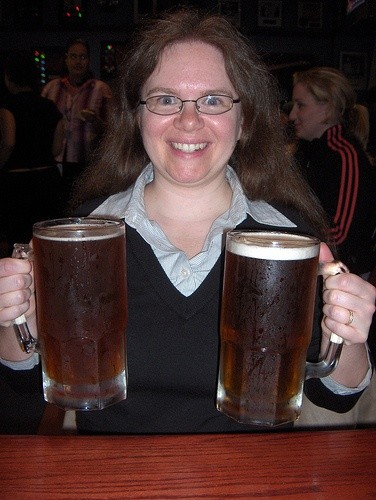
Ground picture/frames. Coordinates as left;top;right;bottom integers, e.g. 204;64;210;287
339;51;369;92
219;0;241;29
258;1;283;28
298;2;323;30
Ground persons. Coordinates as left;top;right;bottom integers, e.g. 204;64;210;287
285;66;376;371
0;7;376;436
40;34;113;186
0;47;67;259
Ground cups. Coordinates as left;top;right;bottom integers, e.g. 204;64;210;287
97;86;113;118
12;216;129;409
214;230;349;426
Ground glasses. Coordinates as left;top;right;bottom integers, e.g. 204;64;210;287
139;95;241;116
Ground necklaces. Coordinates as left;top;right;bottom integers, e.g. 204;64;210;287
64;82;83;120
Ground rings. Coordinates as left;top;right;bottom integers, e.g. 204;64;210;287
345;309;354;326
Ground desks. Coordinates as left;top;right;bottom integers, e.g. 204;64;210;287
0;429;376;500
